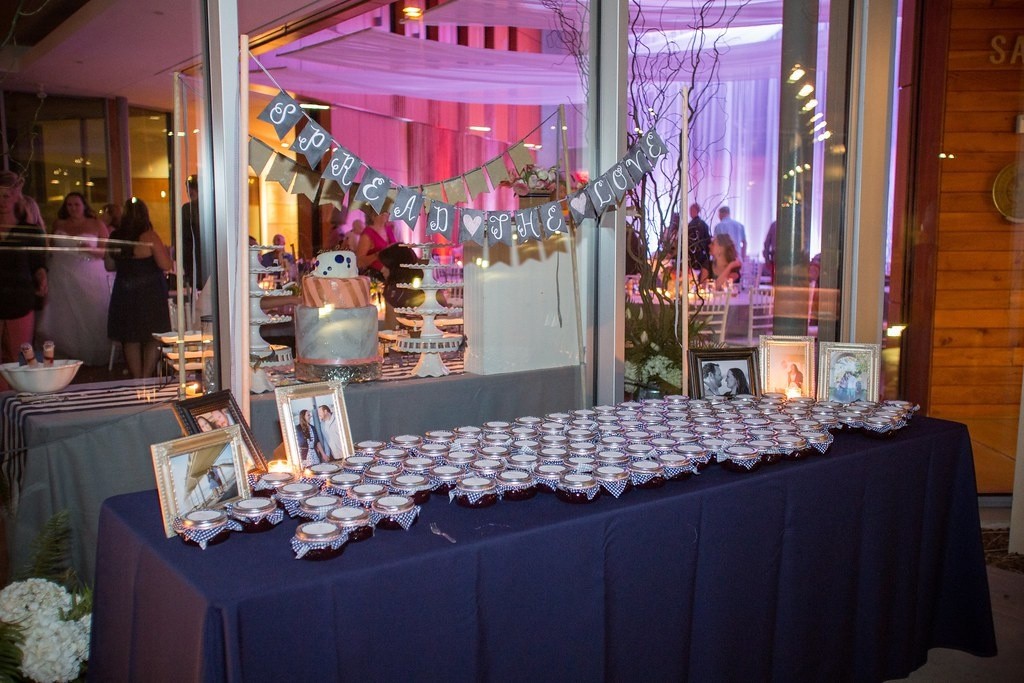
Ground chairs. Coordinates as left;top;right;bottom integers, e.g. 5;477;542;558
748;287;774;343
686;288;731;347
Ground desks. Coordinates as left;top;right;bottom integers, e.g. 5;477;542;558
1;364;585;584
89;417;997;683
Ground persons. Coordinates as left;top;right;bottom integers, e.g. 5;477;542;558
211;410;235;428
0;171;201;379
659;212;679;258
686;204;711;270
296;410;330;468
197;416;217;433
788;364;803;388
700;234;741;287
836;372;857;401
207;465;225;499
341;198;448;336
774;360;791;393
763;221;775;284
713;206;747;268
249;234;294;289
723;368;749;396
316;406;342;461
703;363;723;396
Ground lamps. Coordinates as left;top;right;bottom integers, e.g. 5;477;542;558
403;0;425;21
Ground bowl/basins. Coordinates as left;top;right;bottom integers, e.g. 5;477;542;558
0;359;83;392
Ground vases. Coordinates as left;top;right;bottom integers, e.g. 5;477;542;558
515;189;554;209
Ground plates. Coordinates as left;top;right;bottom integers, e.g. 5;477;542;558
992;161;1024;220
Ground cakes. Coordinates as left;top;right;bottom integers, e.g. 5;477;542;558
293;248;379;364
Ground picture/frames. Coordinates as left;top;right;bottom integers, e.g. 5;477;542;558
758;334;816;401
150;423;251;538
171;389;268;475
688;345;762;400
817;341;881;404
275;379;356;474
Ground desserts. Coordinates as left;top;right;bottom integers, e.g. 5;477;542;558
409;321;421;338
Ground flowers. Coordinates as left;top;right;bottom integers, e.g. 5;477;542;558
624;352;684;404
498;162;589;196
1;504;94;683
